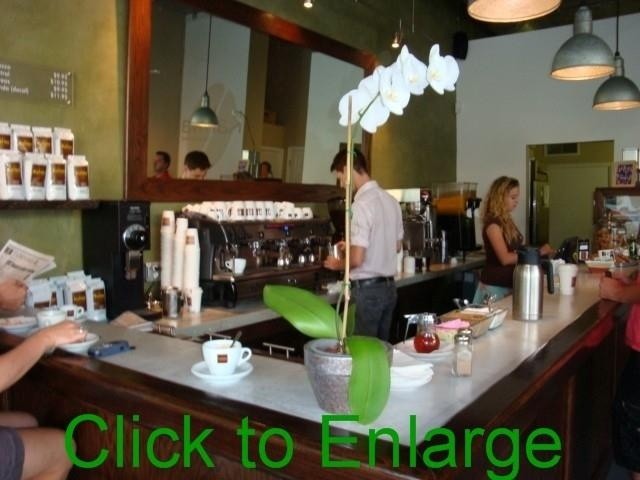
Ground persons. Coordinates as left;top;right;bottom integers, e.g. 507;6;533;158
595;256;639;480
177;151;212;180
471;176;557;307
255;161;274;180
0;317;90;480
322;147;409;341
0;278;28;312
151;150;174;178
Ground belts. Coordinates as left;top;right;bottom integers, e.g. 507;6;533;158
352;276;394;287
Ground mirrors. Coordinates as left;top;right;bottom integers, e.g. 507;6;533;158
121;1;377;201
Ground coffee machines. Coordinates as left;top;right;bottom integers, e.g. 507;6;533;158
385;188;443;265
76;200;164;324
180;210;336;311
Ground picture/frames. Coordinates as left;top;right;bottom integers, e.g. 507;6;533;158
612;160;636;188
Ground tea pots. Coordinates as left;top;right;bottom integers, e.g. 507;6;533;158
510;243;555;323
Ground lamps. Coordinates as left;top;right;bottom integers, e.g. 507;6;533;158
189;0;220;128
464;1;565;24
390;17;406;48
548;1;616;81
591;1;640;113
303;0;317;8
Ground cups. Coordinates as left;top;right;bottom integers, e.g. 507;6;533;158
201;338;251;375
181;312;202;328
396;244;416;274
35;309;66;328
327;244;343;262
224;256;247;277
159;210;203;315
57;304;83;321
182;199;313;223
559;263;579;299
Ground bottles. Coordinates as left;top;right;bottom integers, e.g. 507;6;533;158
585;233;638;269
451;327;478;377
412;311;442;353
164;287;179;319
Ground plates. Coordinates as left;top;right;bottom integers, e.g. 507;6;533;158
1;316;37;332
189;360;257;382
61;330;99;351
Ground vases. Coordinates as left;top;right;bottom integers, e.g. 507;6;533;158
299;336;395;416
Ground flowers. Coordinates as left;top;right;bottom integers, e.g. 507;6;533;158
261;43;463;427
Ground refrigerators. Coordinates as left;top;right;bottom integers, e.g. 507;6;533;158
530;159;551;246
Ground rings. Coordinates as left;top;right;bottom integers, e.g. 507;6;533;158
78;327;84;335
